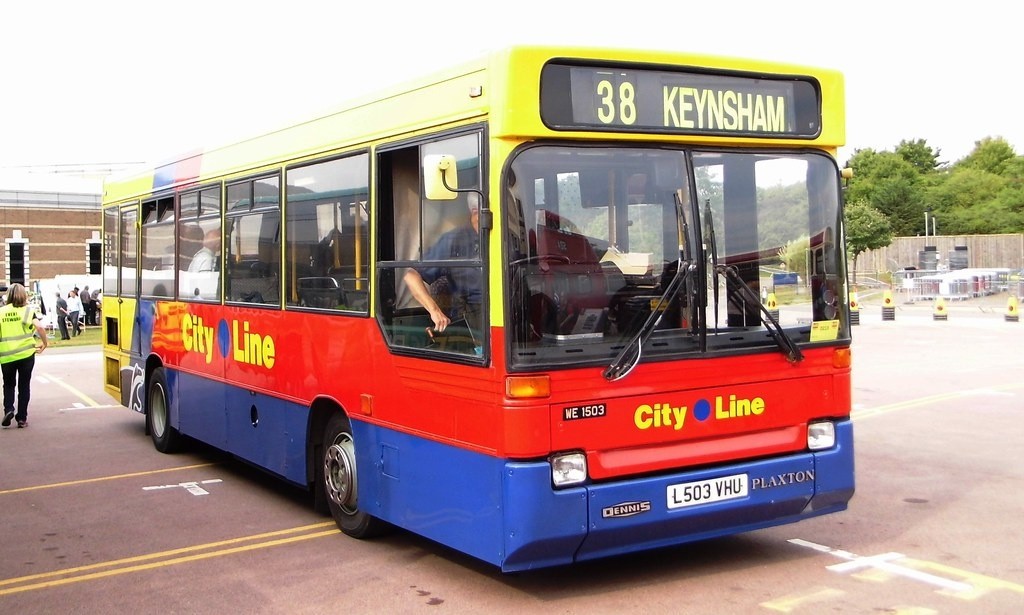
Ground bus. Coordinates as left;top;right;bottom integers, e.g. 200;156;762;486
101;46;854;573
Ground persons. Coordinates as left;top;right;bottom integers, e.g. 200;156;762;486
404;183;535;342
68;285;102;326
187;229;221;273
55;292;70;340
0;283;48;429
67;291;84;337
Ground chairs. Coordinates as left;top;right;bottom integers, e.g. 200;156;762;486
153;261;368;311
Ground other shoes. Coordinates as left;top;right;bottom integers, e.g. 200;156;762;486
1;411;14;427
18;421;28;428
